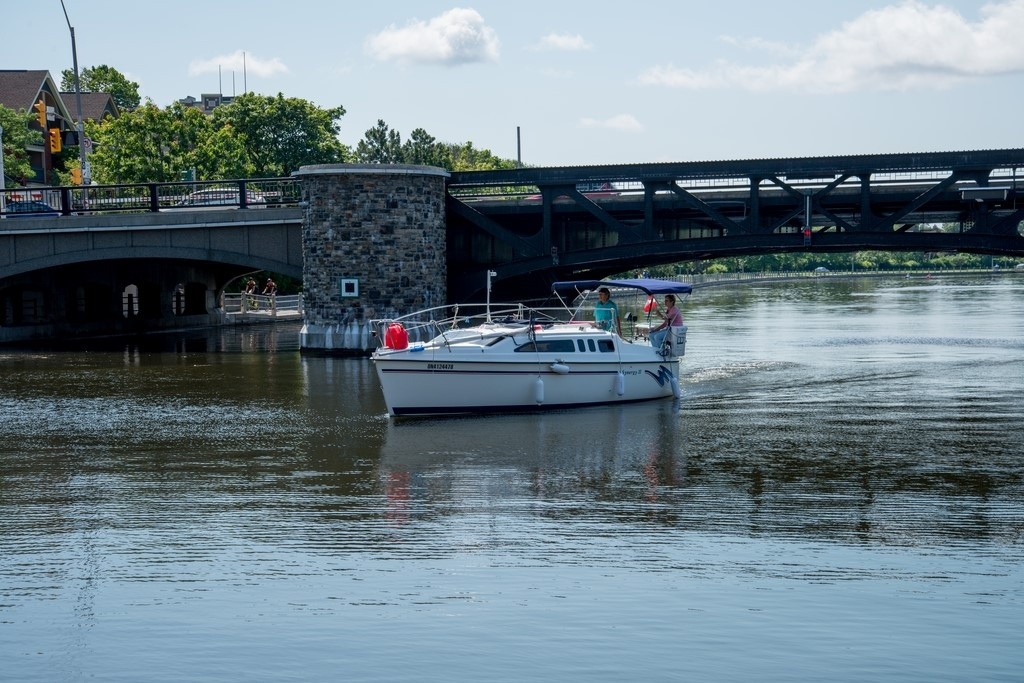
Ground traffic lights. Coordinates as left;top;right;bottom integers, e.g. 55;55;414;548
61;131;79;145
804;227;811;246
49;128;62;153
33;99;46;128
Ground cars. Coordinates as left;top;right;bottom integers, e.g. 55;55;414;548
814;267;829;273
6;201;60;218
523;182;621;200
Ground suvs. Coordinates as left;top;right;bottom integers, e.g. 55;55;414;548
173;187;268;206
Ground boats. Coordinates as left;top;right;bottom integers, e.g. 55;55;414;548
368;269;693;417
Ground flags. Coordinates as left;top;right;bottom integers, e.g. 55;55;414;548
641;272;657;312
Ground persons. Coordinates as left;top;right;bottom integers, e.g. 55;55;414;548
592;288;622;338
648;295;683;348
